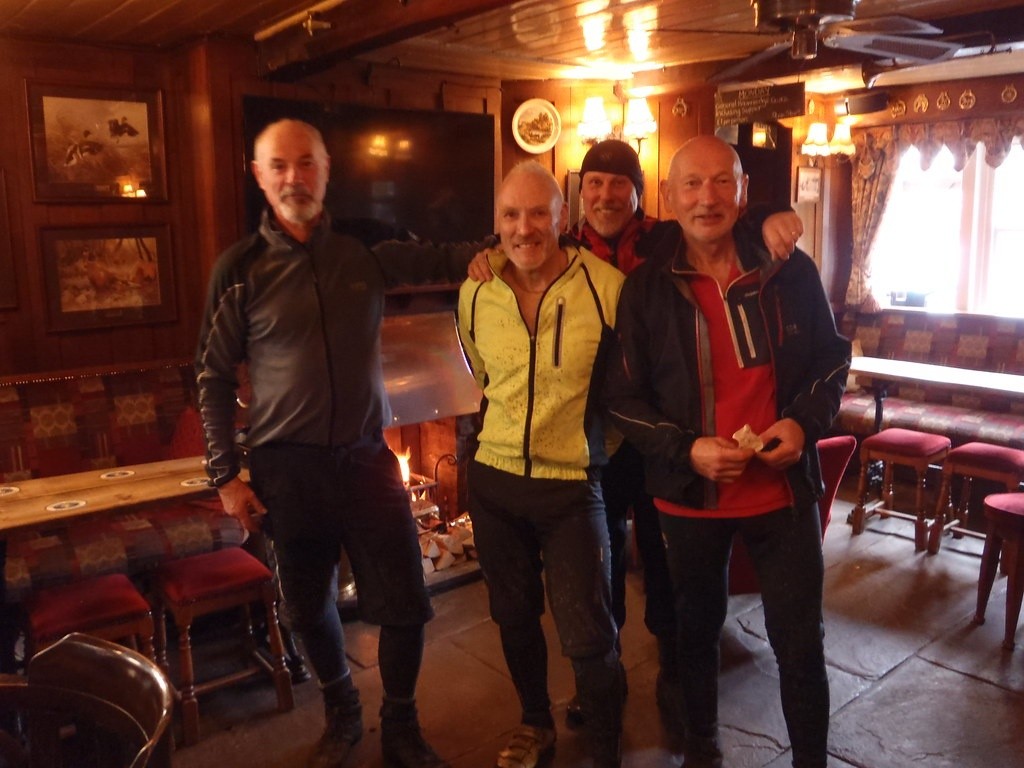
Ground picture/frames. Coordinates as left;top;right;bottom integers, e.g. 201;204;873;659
17;73;172;206
512;98;562;154
0;168;20;309
34;220;186;336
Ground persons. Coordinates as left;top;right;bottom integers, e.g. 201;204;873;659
197;118;592;768
468;141;804;741
602;136;851;768
453;160;628;768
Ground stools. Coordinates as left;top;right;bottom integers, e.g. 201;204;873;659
971;492;1024;650
148;550;297;746
849;426;954;550
924;440;1024;574
21;573;174;754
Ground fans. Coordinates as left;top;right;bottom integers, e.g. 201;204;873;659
641;0;965;85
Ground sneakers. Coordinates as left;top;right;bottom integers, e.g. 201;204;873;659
498;724;556;768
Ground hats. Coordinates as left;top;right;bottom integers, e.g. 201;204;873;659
579;139;645;198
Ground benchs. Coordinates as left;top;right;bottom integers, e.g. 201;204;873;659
832;308;1024;482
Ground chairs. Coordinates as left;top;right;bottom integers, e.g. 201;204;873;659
0;628;173;768
730;428;864;598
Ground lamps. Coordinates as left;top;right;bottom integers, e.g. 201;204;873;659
800;118;856;167
576;90;659;157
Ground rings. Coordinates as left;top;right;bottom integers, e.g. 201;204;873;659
791;230;798;235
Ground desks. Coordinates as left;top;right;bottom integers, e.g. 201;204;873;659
849;356;1024;499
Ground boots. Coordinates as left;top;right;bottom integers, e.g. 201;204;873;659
378;694;449;768
306;668;363;768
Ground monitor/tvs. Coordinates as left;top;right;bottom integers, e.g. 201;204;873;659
243;93;495;292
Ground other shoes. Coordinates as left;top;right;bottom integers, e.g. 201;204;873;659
566;695;583;718
654;676;688;727
